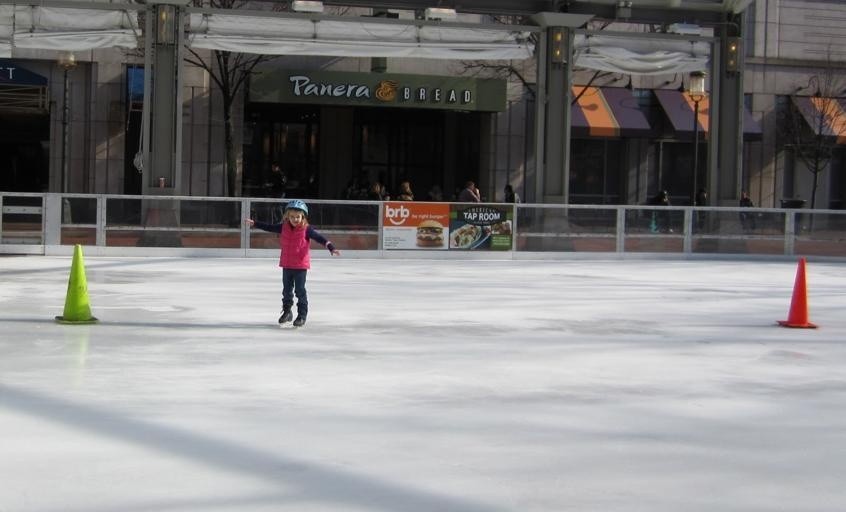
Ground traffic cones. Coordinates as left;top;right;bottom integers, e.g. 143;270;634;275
55;244;99;324
777;256;816;328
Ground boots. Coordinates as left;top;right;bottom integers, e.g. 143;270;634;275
278;303;292;322
293;304;306;326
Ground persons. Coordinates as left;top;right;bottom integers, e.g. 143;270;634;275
644;189;674;234
457;180;481;203
739;188;757;231
244;199;342;329
264;162;287;222
503;184;515;202
341;178;443;203
695;185;707;227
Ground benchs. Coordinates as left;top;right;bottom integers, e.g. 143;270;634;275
569;208;781;233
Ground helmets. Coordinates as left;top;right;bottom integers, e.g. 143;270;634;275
284;199;309;216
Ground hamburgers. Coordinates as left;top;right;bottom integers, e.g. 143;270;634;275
416;220;444;246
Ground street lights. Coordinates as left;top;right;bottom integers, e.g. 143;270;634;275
58;51;77;224
689;71;706;234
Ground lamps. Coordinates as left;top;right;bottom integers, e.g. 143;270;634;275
292;1;324;13
154;5;176;46
424;8;457;21
724;40;742;74
546;26;569;71
